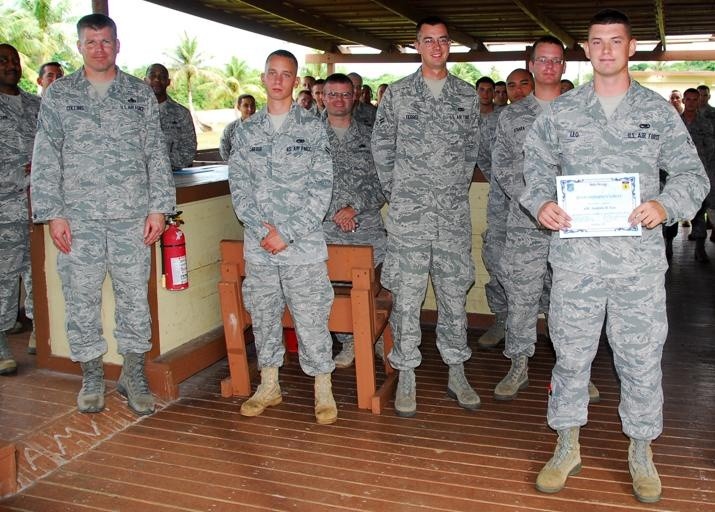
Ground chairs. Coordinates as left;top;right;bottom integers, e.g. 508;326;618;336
220;234;395;416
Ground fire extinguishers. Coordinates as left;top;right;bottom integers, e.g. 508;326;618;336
161;210;189;291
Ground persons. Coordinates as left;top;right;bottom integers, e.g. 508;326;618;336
474;71;554;352
313;71;395;369
493;34;598;402
228;50;338;427
141;62;198;172
29;14;177;412
515;11;712;503
220;95;257;161
372;15;483;418
297;70;715;243
34;60;67;92
0;44;41;376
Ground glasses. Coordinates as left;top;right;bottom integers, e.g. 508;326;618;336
327;91;352;99
536;58;564;65
425;39;449;46
